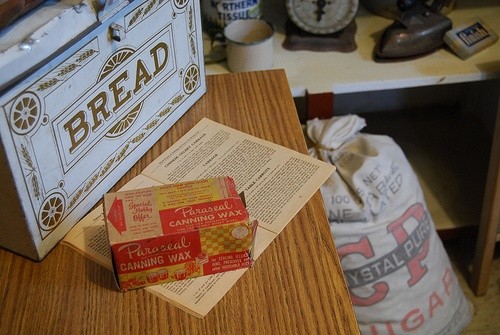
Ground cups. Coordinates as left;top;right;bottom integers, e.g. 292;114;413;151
223;18;275;73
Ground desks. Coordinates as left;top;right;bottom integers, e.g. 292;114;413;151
0;66;358;335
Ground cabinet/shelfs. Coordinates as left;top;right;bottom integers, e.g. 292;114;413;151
201;0;500;300
